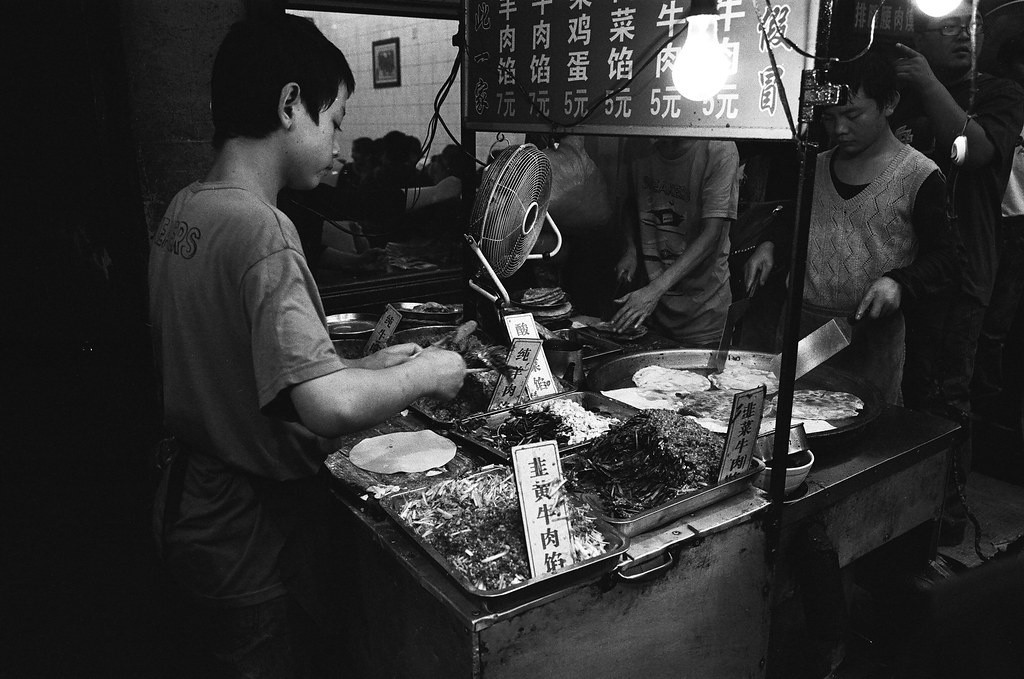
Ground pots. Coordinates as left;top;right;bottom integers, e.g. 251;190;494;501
588;348;884;450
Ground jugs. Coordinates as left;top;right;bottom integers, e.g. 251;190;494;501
544;339;585;393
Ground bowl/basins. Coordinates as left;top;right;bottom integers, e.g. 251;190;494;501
760;450;814;496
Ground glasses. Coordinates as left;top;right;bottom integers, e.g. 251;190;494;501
919;25;985;36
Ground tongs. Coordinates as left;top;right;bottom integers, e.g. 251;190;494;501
408;320;489;373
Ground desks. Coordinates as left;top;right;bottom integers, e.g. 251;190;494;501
268;249;963;679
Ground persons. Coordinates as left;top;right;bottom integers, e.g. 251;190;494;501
894;4;1024;546
615;137;740;349
149;15;466;679
744;50;946;405
337;131;460;232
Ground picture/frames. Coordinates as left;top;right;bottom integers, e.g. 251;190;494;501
372;36;401;89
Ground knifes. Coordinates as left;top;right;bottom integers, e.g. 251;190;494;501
601;272;635;323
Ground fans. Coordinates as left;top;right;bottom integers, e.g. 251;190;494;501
462;142;554;338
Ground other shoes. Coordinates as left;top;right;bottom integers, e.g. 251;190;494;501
938;515;967;545
